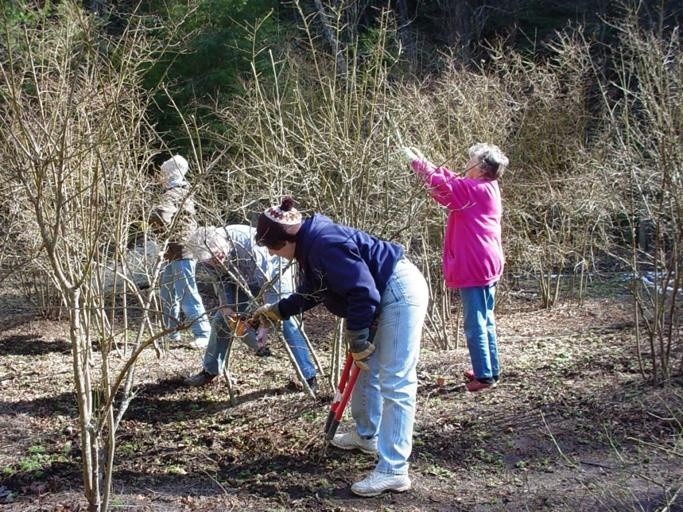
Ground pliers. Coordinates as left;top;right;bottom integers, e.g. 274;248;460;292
317;320;379;465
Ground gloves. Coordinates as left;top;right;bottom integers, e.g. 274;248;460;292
252;300;286;328
345;327;377;371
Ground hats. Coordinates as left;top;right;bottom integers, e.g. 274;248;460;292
160;154;189;183
257;197;303;244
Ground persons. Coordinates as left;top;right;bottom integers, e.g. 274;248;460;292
133;154;212;350
176;219;317;392
244;200;432;498
397;138;510;392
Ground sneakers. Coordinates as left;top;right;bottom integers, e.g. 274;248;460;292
329;431;378;455
182;370;218;387
306;375;319;391
464;365;501;392
351;470;412;497
160;336;181;343
188;337;209;348
